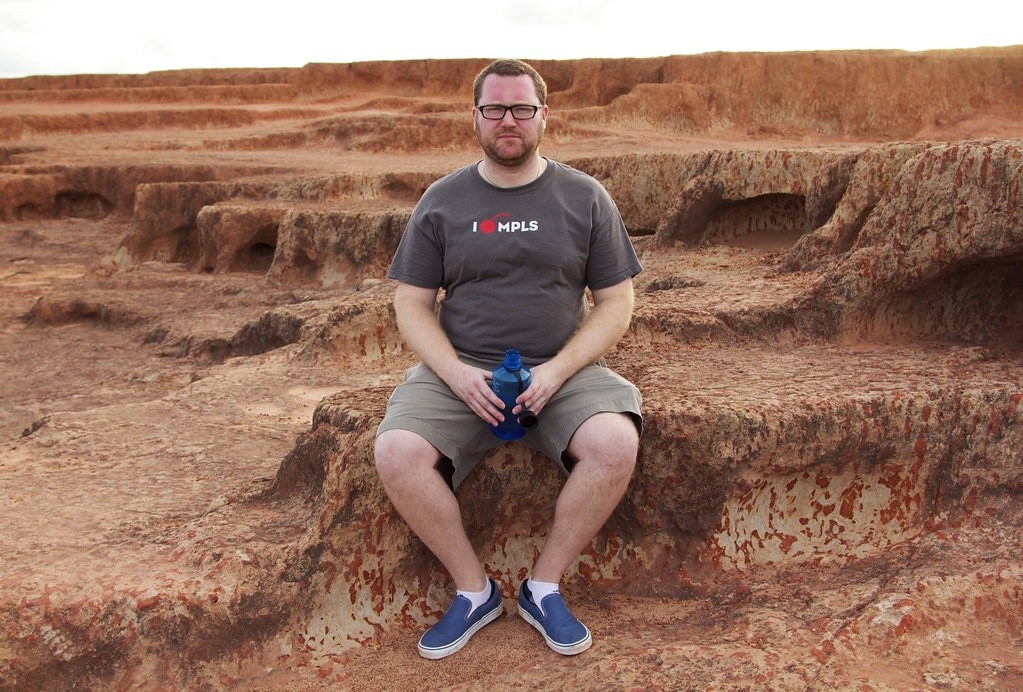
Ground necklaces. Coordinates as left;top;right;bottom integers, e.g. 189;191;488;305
483;159;541;188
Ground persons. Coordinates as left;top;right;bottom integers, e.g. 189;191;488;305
374;58;643;659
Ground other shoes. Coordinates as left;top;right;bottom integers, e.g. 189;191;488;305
417;578;504;659
517;579;592;655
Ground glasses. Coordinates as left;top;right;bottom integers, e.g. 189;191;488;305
476;104;543;120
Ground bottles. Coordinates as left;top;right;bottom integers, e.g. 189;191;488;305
491;349;532;440
